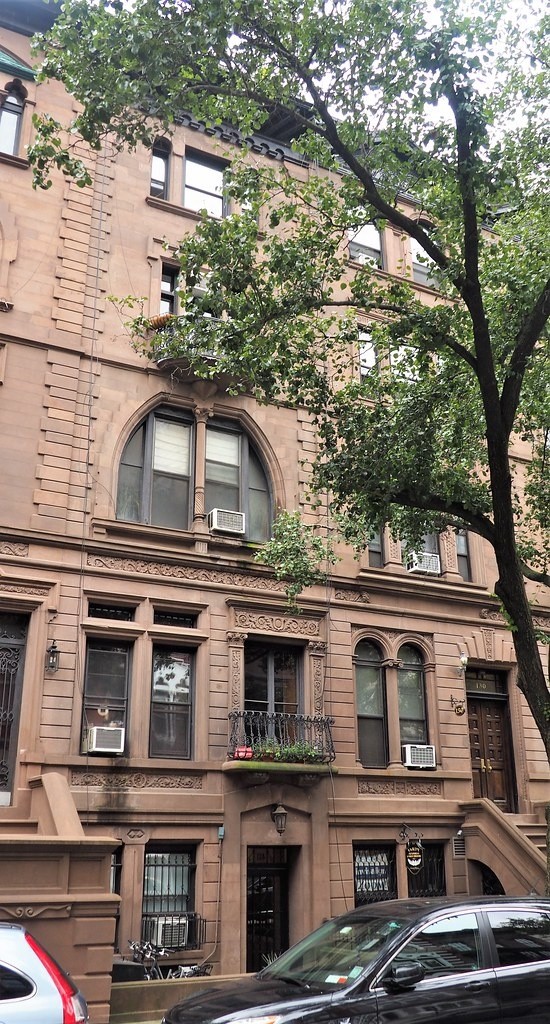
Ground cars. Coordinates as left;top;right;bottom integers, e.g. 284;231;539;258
0;920;90;1024
154;894;550;1024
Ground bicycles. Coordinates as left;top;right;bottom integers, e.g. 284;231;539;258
126;939;214;982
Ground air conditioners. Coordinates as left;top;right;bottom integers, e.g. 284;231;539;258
406;551;441;575
208;508;246;534
143;916;187;947
89;726;125;753
401;744;436;768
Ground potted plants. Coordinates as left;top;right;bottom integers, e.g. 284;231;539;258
251;737;278;762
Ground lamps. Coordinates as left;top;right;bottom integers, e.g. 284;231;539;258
456;651;468;677
270;802;288;836
45;638;62;672
